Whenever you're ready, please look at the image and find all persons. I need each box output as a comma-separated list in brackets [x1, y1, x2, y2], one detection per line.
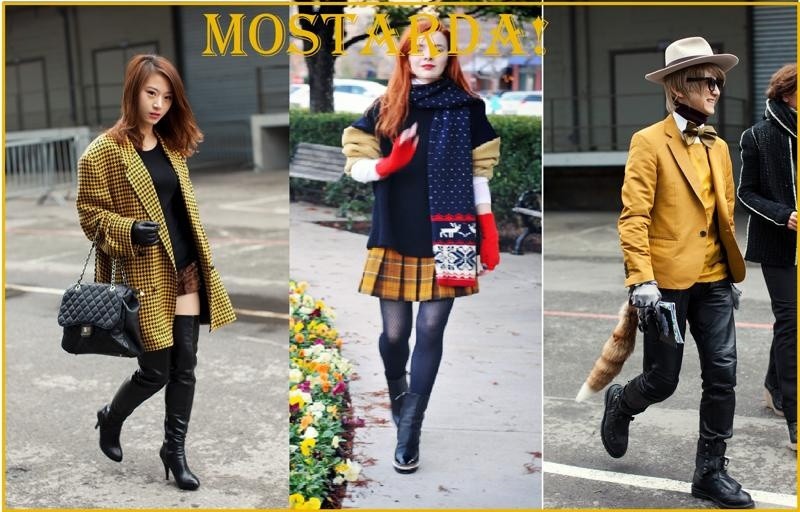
[466, 74, 481, 95]
[600, 35, 757, 508]
[339, 16, 504, 475]
[75, 51, 239, 492]
[736, 60, 797, 453]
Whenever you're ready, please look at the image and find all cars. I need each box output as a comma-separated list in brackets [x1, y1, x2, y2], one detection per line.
[290, 77, 542, 120]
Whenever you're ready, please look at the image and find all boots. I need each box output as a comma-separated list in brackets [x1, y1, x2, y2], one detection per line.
[692, 438, 754, 507]
[601, 376, 647, 460]
[381, 369, 429, 475]
[95, 313, 202, 492]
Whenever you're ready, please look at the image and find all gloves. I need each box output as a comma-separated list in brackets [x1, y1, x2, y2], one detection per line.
[132, 219, 166, 248]
[632, 282, 661, 333]
[474, 212, 501, 272]
[377, 130, 418, 179]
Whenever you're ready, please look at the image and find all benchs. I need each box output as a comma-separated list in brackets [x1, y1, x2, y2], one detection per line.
[289, 139, 351, 209]
[509, 189, 542, 251]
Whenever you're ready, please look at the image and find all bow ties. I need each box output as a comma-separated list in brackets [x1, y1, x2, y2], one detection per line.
[682, 120, 718, 149]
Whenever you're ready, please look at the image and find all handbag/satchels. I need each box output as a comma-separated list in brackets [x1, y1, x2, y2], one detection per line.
[57, 282, 145, 358]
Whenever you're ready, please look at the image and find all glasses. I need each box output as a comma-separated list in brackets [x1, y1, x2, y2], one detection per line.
[685, 74, 725, 91]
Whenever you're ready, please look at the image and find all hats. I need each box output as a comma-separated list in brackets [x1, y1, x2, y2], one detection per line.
[645, 35, 739, 84]
